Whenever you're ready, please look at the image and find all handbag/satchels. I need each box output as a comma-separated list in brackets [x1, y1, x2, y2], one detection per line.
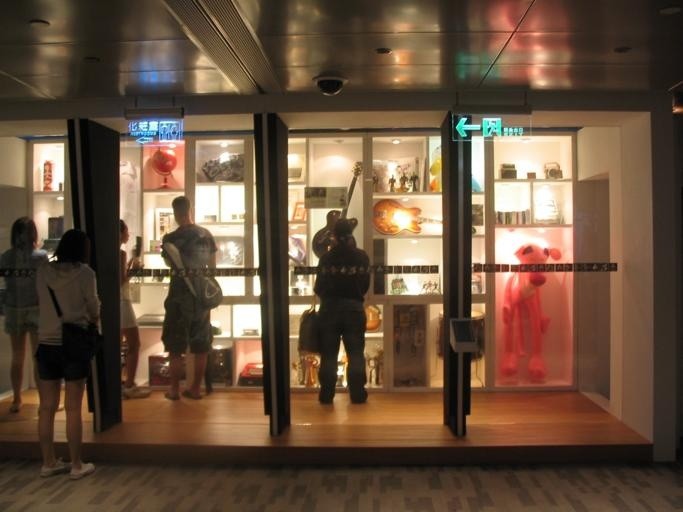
[297, 308, 321, 355]
[198, 273, 225, 309]
[59, 321, 101, 360]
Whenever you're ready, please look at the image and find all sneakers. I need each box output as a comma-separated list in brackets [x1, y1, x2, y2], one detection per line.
[121, 385, 152, 401]
[69, 463, 95, 480]
[39, 460, 70, 478]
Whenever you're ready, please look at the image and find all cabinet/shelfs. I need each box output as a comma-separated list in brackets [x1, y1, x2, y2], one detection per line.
[307, 136, 441, 301]
[246, 138, 313, 302]
[368, 131, 491, 396]
[116, 140, 143, 303]
[287, 299, 446, 394]
[488, 130, 577, 393]
[188, 138, 252, 300]
[141, 143, 196, 285]
[135, 285, 265, 395]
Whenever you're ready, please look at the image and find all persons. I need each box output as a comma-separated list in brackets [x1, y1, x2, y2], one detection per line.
[0, 216, 50, 415]
[311, 218, 370, 403]
[32, 227, 102, 480]
[118, 218, 153, 396]
[160, 196, 218, 402]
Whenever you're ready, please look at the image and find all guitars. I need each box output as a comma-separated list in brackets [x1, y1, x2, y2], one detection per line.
[365, 304, 380, 329]
[312, 162, 363, 258]
[371, 199, 476, 237]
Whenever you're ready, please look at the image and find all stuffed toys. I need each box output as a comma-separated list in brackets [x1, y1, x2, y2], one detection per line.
[500, 234, 561, 380]
